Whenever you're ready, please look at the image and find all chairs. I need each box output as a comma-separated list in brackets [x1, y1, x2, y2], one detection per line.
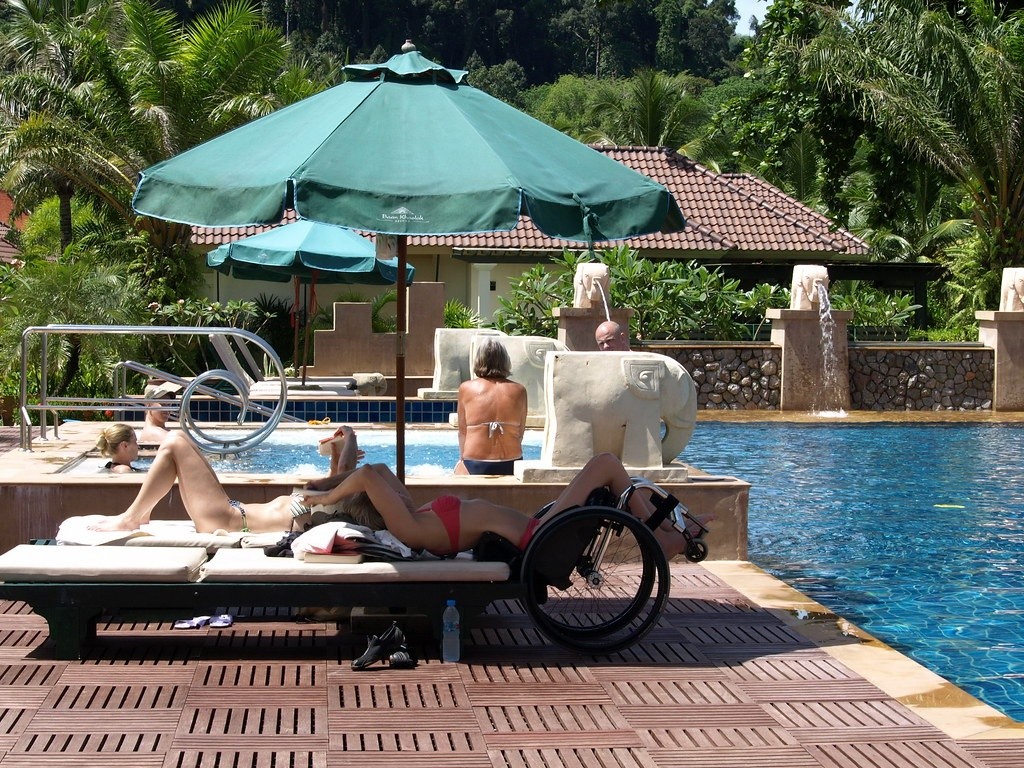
[231, 327, 357, 384]
[209, 332, 357, 396]
[216, 332, 348, 391]
[220, 333, 351, 388]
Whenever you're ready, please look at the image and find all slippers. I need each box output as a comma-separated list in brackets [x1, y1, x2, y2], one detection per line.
[209, 614, 232, 627]
[174, 616, 210, 628]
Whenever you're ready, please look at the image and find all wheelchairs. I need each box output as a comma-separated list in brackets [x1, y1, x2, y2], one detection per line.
[475, 478, 710, 652]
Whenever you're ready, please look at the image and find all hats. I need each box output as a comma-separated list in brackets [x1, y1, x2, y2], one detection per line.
[145, 388, 176, 399]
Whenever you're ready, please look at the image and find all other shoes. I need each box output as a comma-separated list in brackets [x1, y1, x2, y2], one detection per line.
[350, 621, 405, 670]
[390, 651, 413, 668]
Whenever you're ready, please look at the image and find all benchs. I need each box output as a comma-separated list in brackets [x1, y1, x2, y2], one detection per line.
[0, 513, 510, 660]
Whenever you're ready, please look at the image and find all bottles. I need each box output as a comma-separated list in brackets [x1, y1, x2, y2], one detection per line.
[442, 600, 460, 662]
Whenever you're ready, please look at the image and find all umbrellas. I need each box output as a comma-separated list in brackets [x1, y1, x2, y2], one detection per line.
[208, 216, 422, 388]
[132, 37, 688, 484]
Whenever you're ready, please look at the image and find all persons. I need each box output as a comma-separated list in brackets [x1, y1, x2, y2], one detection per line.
[298, 449, 720, 557]
[86, 424, 365, 534]
[96, 422, 140, 474]
[595, 320, 634, 351]
[452, 337, 528, 475]
[138, 384, 176, 442]
